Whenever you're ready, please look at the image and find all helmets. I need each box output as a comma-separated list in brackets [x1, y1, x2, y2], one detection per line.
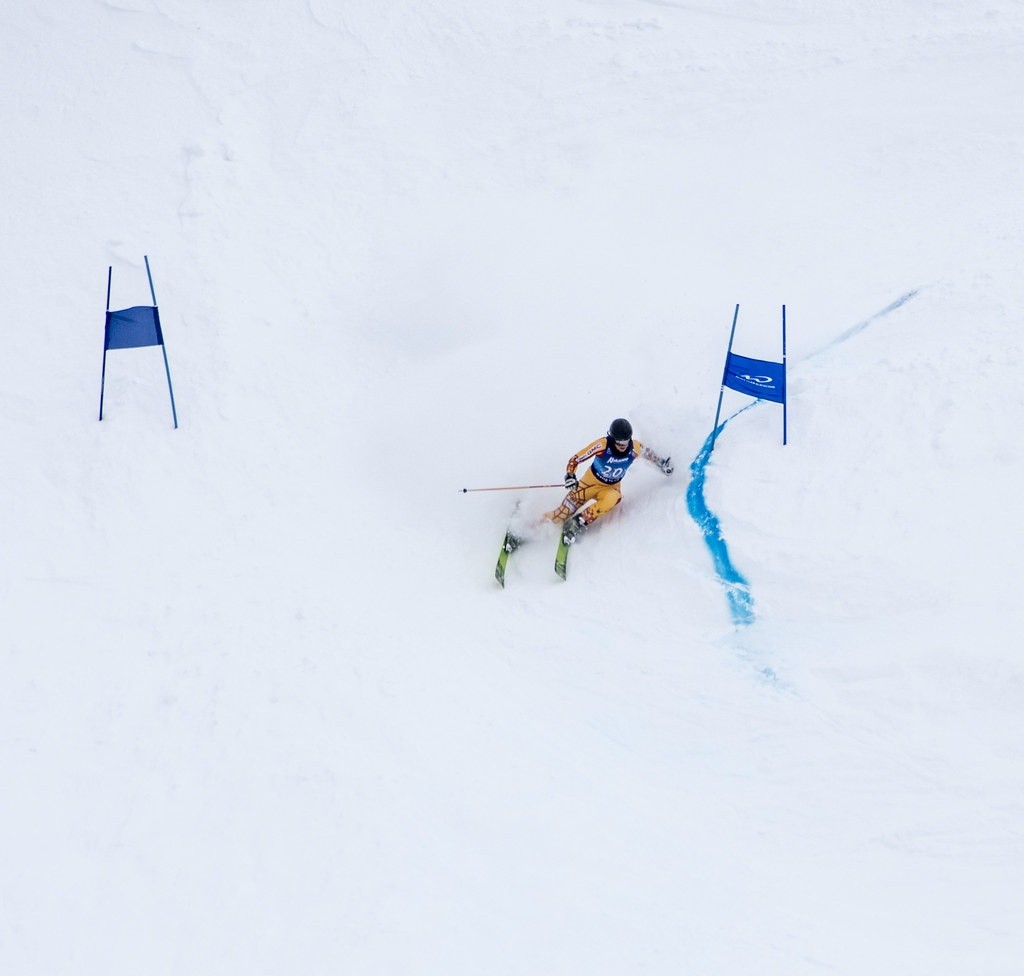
[607, 418, 633, 442]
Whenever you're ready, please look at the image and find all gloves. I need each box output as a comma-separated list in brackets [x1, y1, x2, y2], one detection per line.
[564, 474, 579, 493]
[661, 463, 674, 475]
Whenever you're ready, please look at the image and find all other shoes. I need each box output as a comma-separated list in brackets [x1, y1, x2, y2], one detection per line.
[509, 534, 518, 548]
[562, 521, 576, 546]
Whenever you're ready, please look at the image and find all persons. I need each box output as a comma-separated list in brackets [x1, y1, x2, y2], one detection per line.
[521, 418, 674, 556]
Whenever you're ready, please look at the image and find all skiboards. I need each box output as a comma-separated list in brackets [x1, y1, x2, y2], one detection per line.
[494, 499, 570, 590]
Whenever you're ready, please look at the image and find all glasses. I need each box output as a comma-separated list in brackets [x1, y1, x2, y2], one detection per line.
[615, 439, 630, 445]
